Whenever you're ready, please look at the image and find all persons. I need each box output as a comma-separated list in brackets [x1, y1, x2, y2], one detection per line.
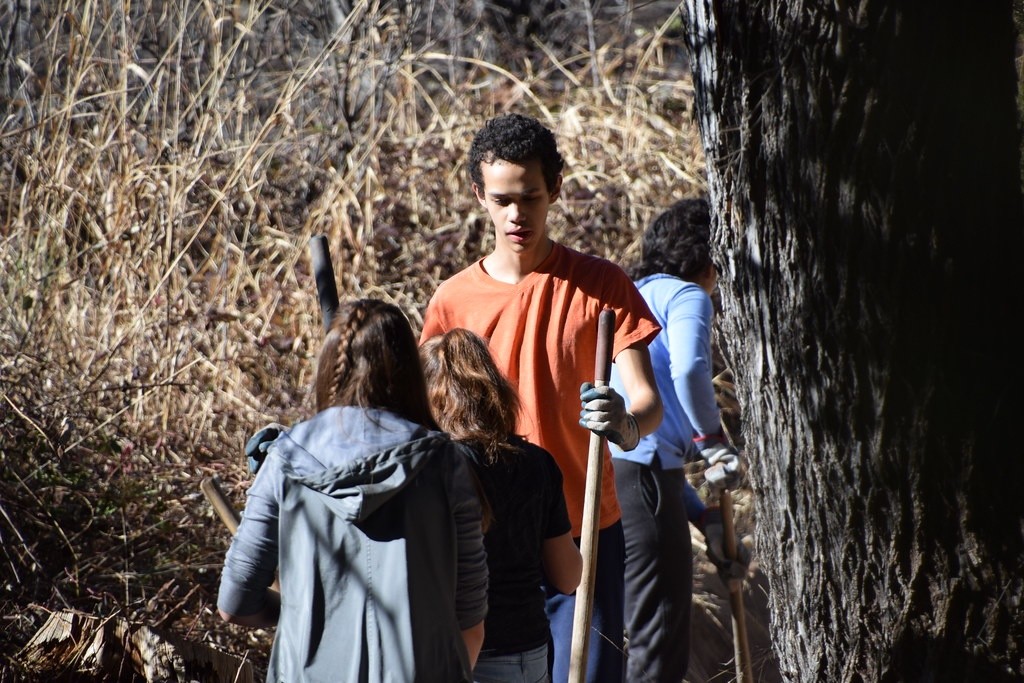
[215, 299, 488, 683]
[417, 114, 664, 683]
[418, 328, 584, 683]
[599, 198, 750, 683]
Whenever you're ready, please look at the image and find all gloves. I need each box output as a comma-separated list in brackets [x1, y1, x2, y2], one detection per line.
[245, 423, 292, 474]
[579, 382, 640, 451]
[692, 424, 744, 492]
